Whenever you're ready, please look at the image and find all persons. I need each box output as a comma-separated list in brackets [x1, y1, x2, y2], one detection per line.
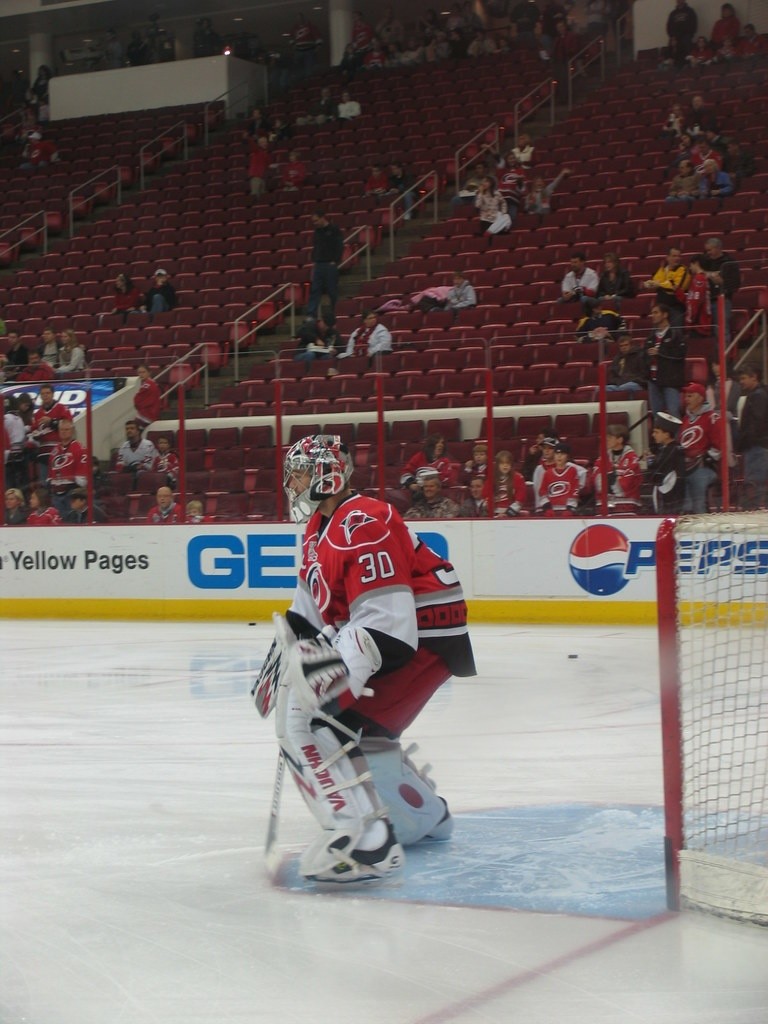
[0, 326, 214, 528]
[242, 87, 361, 198]
[295, 209, 477, 360]
[663, 96, 755, 202]
[252, 433, 477, 882]
[666, 0, 768, 63]
[95, 269, 177, 316]
[400, 357, 768, 520]
[361, 161, 418, 221]
[450, 132, 571, 238]
[558, 238, 742, 449]
[0, 12, 323, 169]
[337, 0, 632, 88]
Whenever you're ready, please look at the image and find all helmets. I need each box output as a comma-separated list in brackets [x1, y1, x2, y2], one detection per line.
[286, 434, 354, 524]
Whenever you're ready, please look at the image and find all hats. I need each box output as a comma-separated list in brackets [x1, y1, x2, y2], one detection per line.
[682, 381, 706, 399]
[654, 412, 682, 433]
[555, 443, 572, 454]
[544, 437, 560, 448]
[155, 269, 167, 276]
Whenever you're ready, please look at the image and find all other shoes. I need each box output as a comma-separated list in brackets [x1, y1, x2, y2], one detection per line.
[382, 796, 454, 842]
[304, 825, 404, 881]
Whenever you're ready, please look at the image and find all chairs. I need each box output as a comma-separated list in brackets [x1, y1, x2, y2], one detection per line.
[0, 32, 768, 526]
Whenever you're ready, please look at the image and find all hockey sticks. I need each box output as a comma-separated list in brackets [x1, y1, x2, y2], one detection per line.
[257, 744, 306, 889]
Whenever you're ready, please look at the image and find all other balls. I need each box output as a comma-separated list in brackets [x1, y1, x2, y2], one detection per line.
[568, 655, 578, 658]
[249, 623, 256, 625]
